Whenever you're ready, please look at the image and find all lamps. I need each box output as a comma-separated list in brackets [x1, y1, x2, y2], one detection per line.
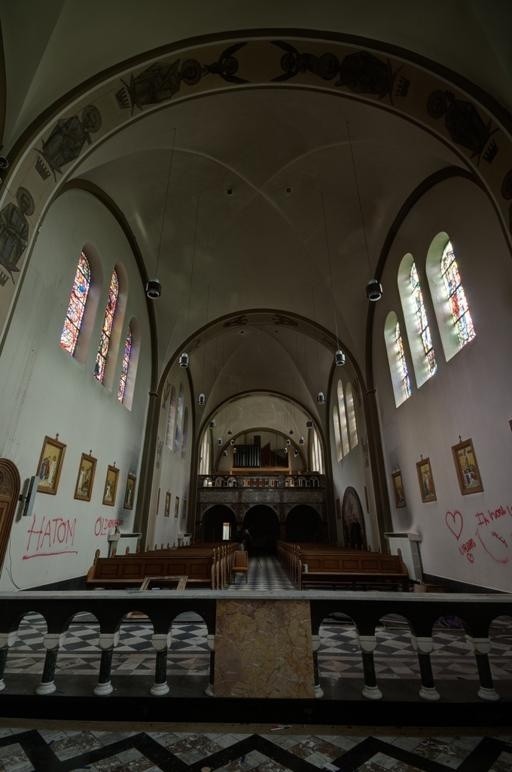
[344, 119, 384, 302]
[19, 476, 39, 517]
[179, 195, 200, 368]
[320, 188, 346, 368]
[146, 128, 176, 300]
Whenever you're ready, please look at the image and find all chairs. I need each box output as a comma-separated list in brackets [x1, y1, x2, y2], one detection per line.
[229, 550, 249, 585]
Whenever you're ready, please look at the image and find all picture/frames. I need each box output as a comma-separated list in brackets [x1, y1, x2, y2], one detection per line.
[392, 469, 406, 509]
[74, 452, 98, 501]
[415, 456, 437, 503]
[36, 435, 67, 496]
[124, 473, 137, 511]
[101, 464, 120, 507]
[451, 437, 484, 496]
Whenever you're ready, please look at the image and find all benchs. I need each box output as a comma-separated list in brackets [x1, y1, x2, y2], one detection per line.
[84, 539, 244, 591]
[278, 539, 413, 591]
